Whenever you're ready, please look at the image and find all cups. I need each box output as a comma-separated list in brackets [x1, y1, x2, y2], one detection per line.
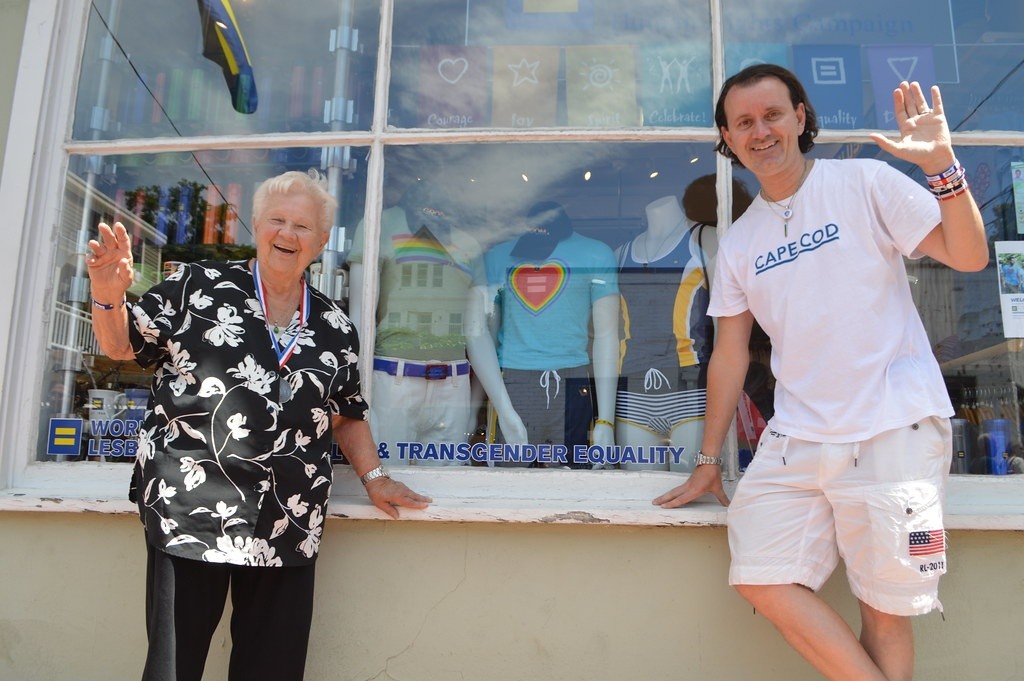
[87, 389, 127, 432]
[112, 183, 243, 262]
[950, 419, 971, 474]
[982, 419, 1011, 475]
[119, 65, 325, 123]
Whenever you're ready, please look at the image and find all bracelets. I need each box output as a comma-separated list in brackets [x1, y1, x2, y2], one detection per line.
[91, 296, 126, 311]
[925, 158, 969, 202]
[595, 420, 615, 432]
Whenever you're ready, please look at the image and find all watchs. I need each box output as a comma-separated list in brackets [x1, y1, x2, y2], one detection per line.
[359, 464, 389, 485]
[693, 449, 724, 467]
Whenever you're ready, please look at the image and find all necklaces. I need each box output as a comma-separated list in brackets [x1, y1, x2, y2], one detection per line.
[643, 218, 683, 268]
[761, 159, 809, 237]
[270, 318, 283, 334]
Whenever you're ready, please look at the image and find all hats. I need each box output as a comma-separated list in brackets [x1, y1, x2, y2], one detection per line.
[398, 177, 451, 247]
[510, 201, 570, 262]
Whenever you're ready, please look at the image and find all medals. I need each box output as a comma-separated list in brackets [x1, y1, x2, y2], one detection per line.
[279, 377, 292, 403]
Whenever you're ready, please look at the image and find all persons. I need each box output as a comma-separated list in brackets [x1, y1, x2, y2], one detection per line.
[613, 195, 718, 472]
[460, 201, 620, 470]
[83, 169, 433, 681]
[650, 63, 991, 681]
[346, 179, 529, 467]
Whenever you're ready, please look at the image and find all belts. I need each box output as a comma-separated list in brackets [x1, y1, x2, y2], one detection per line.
[373, 358, 470, 380]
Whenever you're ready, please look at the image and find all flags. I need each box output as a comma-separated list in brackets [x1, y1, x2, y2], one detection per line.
[197, 0, 258, 115]
